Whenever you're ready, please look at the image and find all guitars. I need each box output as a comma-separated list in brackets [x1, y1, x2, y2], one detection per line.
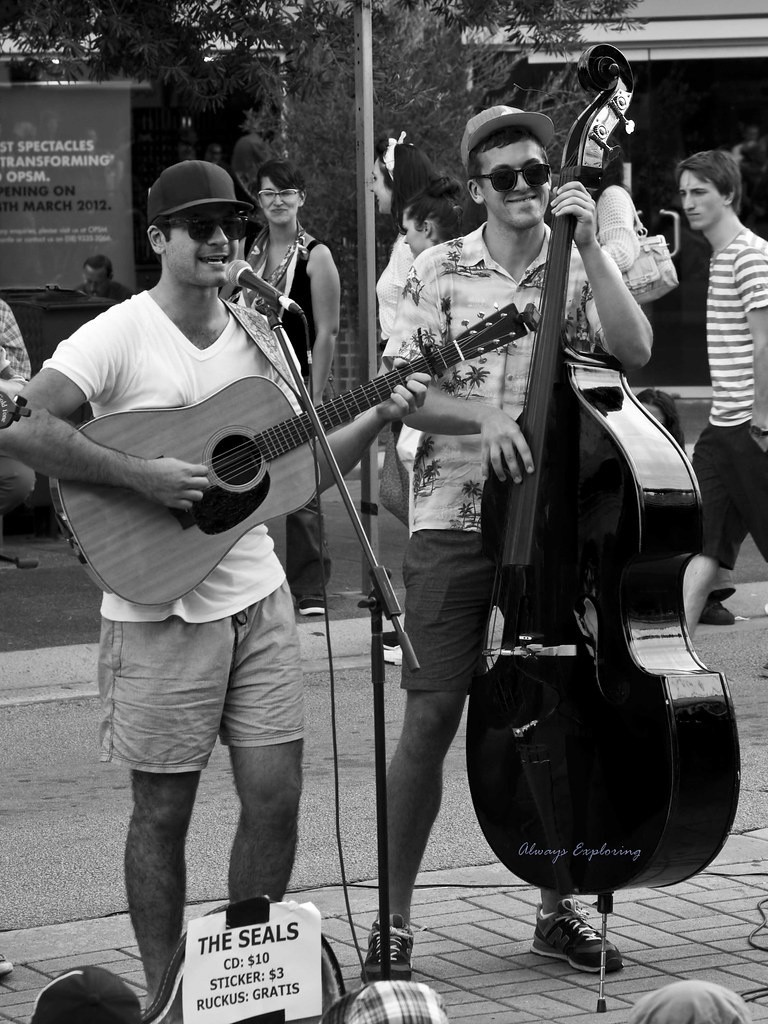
[50, 298, 529, 609]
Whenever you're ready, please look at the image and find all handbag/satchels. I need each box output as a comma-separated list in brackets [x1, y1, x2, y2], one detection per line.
[593, 185, 680, 305]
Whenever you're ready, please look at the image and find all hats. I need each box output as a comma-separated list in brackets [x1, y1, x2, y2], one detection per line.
[459, 105, 554, 168]
[145, 159, 254, 226]
[319, 980, 447, 1024]
[30, 965, 141, 1023]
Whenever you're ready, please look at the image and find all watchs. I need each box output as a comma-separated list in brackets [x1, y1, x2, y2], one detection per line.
[748, 423, 768, 438]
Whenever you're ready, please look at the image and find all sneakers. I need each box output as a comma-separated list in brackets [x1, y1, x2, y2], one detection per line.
[362, 914, 414, 985]
[530, 899, 624, 973]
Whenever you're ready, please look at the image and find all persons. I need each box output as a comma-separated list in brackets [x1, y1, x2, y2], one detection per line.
[319, 980, 449, 1024]
[636, 389, 736, 625]
[178, 130, 284, 188]
[627, 980, 752, 1024]
[669, 119, 768, 385]
[580, 136, 678, 305]
[220, 159, 341, 616]
[676, 150, 768, 642]
[0, 298, 36, 515]
[0, 954, 14, 975]
[368, 137, 466, 666]
[360, 105, 653, 981]
[0, 159, 431, 1024]
[77, 255, 132, 302]
[30, 965, 141, 1024]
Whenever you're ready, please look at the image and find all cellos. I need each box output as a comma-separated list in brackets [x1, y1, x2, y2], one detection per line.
[466, 43, 744, 1011]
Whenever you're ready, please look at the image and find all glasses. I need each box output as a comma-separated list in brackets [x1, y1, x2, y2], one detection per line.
[470, 162, 550, 192]
[258, 188, 300, 200]
[160, 213, 248, 241]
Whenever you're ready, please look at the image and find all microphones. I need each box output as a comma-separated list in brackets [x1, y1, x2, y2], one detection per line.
[226, 260, 305, 317]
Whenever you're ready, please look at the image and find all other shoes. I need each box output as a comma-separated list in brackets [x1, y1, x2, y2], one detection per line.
[299, 600, 326, 615]
[702, 599, 735, 626]
